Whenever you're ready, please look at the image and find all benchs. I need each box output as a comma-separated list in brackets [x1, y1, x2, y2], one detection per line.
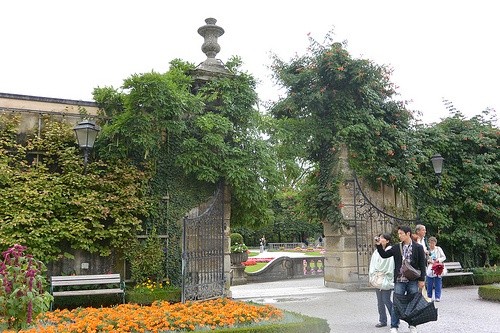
[442, 261, 475, 285]
[49, 273, 127, 313]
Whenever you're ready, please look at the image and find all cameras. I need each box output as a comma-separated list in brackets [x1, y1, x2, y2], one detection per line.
[374, 237, 380, 242]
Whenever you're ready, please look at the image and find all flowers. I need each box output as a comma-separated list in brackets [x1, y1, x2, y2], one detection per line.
[431, 261, 444, 277]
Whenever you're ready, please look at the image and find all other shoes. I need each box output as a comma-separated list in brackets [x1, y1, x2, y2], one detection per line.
[410, 326, 417, 333]
[435, 298, 440, 302]
[391, 328, 399, 333]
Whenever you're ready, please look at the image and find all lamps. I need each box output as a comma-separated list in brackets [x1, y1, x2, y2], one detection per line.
[430, 152, 445, 178]
[72, 119, 100, 175]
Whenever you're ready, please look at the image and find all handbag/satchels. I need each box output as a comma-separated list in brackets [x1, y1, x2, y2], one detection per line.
[370, 271, 390, 291]
[400, 242, 421, 281]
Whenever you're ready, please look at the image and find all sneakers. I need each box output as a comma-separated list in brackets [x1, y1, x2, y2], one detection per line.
[375, 322, 387, 327]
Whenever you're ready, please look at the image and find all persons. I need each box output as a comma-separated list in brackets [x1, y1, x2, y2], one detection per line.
[369, 233, 395, 327]
[411, 224, 429, 258]
[425, 237, 447, 302]
[374, 225, 426, 333]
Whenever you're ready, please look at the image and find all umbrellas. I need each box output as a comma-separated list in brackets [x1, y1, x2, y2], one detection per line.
[431, 262, 444, 275]
[393, 288, 438, 328]
